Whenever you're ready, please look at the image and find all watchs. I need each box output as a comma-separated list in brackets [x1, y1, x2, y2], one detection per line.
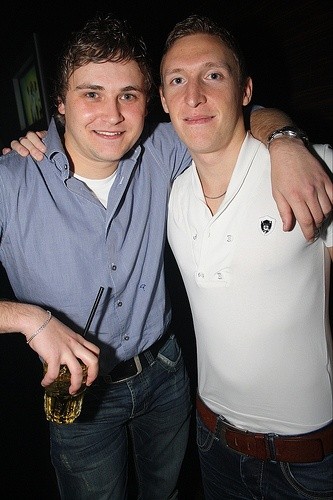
[266, 126, 310, 149]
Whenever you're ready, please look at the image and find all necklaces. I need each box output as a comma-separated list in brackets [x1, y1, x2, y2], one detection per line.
[204, 192, 226, 199]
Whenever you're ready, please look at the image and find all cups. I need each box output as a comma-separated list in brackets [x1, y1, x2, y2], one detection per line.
[43, 361, 89, 424]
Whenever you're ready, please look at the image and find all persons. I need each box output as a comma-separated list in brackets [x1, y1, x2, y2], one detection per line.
[0, 16, 333, 500]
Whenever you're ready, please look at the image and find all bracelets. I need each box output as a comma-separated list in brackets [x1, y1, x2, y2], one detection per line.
[27, 311, 51, 344]
